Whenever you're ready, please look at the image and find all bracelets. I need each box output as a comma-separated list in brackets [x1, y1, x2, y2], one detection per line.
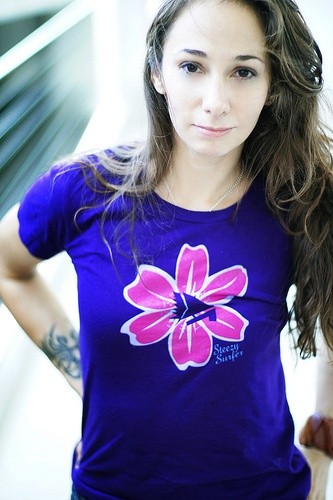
[297, 414, 333, 458]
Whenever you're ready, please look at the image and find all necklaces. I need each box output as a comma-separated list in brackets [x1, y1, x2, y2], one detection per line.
[152, 157, 247, 213]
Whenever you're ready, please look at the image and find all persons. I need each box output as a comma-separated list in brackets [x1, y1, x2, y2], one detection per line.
[0, 0, 333, 500]
